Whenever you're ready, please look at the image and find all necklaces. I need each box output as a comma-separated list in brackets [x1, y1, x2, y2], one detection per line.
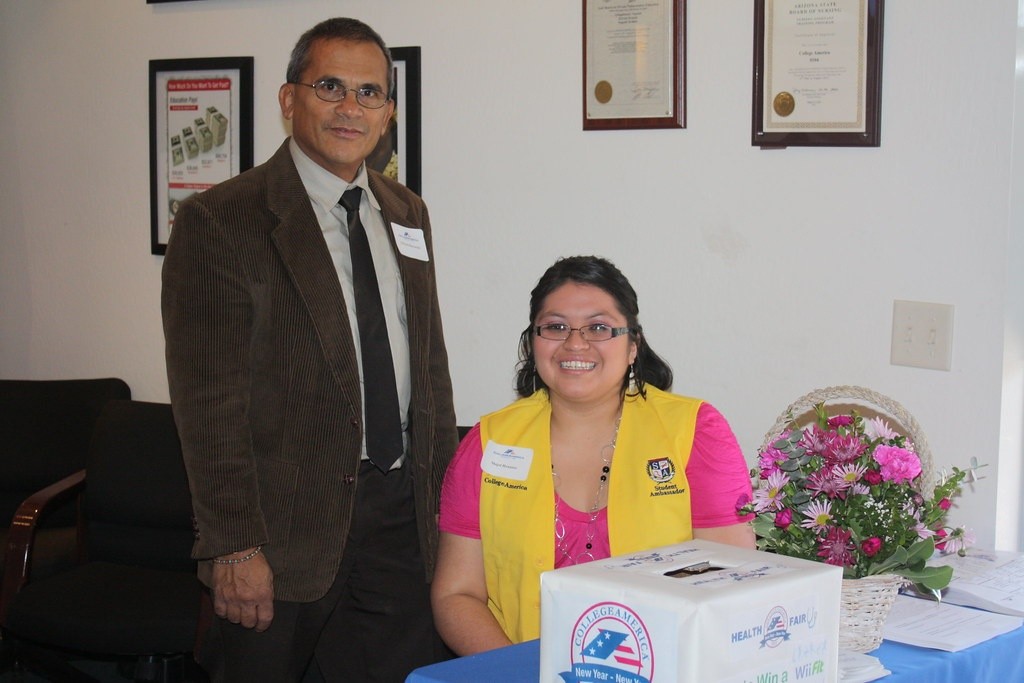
[551, 415, 621, 565]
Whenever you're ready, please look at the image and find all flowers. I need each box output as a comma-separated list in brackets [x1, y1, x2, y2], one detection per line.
[732, 397, 993, 604]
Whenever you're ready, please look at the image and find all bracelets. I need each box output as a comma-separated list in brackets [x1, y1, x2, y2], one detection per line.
[213, 546, 262, 564]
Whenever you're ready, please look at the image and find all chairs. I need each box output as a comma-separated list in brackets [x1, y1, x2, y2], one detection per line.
[0, 375, 132, 582]
[0, 396, 241, 683]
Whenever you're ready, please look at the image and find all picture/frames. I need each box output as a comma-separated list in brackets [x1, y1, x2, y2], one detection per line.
[365, 45, 422, 201]
[750, 0, 884, 150]
[147, 55, 256, 257]
[579, 0, 687, 130]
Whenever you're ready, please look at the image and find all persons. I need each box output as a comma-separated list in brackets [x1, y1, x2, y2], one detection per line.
[430, 255, 755, 657]
[157, 18, 461, 682]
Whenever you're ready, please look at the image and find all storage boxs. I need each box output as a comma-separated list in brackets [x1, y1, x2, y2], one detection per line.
[536, 539, 847, 682]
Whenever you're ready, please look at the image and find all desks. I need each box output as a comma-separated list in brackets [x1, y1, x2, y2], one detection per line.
[405, 542, 1023, 682]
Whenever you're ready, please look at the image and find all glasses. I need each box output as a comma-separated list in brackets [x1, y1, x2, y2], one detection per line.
[287, 78, 392, 109]
[530, 322, 630, 342]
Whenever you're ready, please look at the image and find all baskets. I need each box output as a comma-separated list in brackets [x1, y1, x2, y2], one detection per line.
[759, 384, 931, 653]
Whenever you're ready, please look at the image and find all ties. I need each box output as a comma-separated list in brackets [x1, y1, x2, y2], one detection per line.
[338, 186, 404, 475]
